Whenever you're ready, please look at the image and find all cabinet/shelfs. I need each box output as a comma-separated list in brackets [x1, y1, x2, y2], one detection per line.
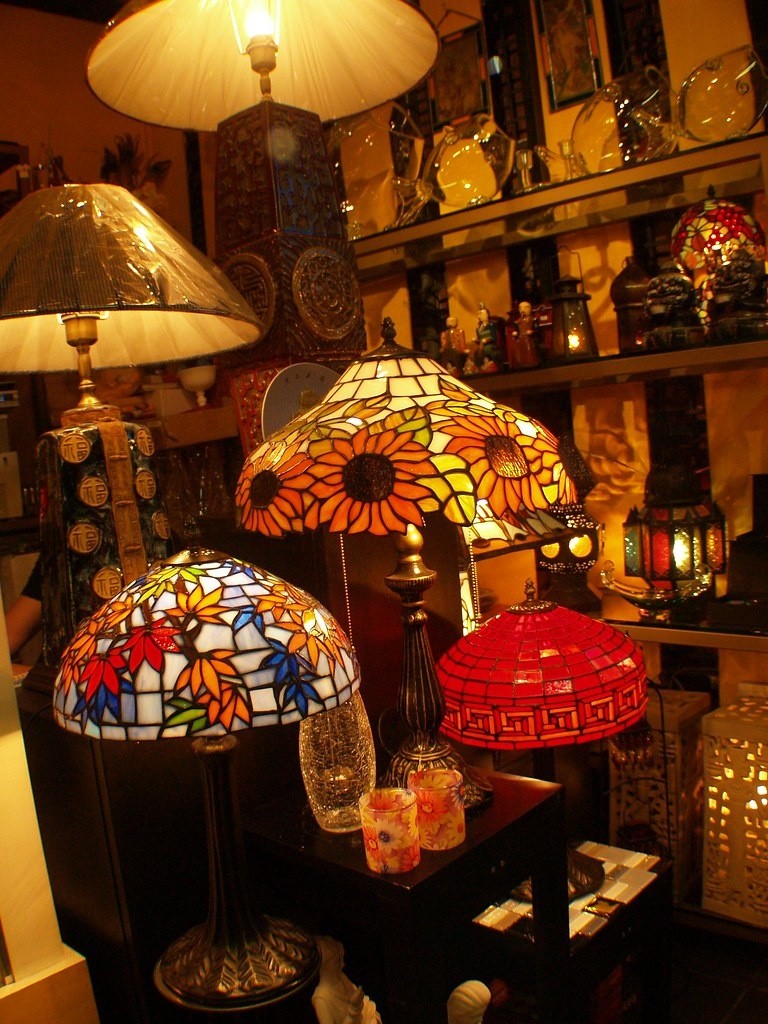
[19, 132, 768, 1024]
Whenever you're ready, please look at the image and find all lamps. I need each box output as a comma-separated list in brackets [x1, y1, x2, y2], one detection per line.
[599, 689, 712, 900]
[701, 680, 768, 930]
[537, 430, 605, 612]
[233, 318, 581, 804]
[436, 580, 651, 904]
[86, 0, 444, 461]
[623, 434, 727, 624]
[0, 184, 265, 673]
[48, 538, 363, 1024]
[670, 183, 768, 341]
[551, 274, 601, 362]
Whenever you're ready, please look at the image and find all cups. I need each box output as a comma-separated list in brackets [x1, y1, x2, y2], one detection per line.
[411, 769, 466, 851]
[358, 788, 420, 874]
